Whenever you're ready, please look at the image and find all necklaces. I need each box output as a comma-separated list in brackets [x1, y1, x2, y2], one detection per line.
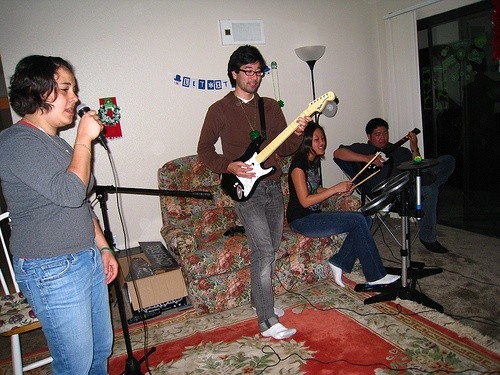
[236, 89, 260, 140]
[22, 118, 70, 153]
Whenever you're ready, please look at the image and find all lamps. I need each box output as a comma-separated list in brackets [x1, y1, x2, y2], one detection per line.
[295, 45, 339, 187]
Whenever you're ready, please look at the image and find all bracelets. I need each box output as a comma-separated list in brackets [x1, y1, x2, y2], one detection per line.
[74, 143, 92, 155]
[100, 248, 115, 255]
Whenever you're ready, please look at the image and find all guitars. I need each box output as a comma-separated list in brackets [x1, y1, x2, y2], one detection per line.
[220, 90, 337, 203]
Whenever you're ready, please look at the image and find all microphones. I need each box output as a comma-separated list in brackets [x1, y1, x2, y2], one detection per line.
[75, 104, 109, 150]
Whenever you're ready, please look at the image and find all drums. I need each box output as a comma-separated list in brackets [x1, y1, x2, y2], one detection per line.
[360, 193, 391, 217]
[371, 171, 416, 197]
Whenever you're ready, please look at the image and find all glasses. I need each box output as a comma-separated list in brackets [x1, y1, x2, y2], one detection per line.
[238, 68, 264, 77]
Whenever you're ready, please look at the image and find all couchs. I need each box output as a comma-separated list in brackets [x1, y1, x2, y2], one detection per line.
[155, 147, 360, 313]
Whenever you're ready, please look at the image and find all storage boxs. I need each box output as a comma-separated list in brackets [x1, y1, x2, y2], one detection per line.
[115, 242, 189, 324]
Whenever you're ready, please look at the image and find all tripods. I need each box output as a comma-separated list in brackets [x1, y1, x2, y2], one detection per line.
[91, 186, 212, 374]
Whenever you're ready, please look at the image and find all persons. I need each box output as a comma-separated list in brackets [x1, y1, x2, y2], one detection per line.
[333, 118, 456, 253]
[0, 55, 120, 375]
[198, 45, 312, 340]
[286, 121, 401, 288]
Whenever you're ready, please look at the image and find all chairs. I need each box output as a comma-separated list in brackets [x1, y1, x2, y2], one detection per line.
[0, 212, 53, 375]
[333, 151, 424, 249]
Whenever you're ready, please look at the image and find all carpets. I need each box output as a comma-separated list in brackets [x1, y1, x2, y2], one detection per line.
[0, 268, 500, 375]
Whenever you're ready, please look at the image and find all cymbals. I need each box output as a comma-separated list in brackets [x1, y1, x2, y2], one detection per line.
[396, 159, 440, 170]
